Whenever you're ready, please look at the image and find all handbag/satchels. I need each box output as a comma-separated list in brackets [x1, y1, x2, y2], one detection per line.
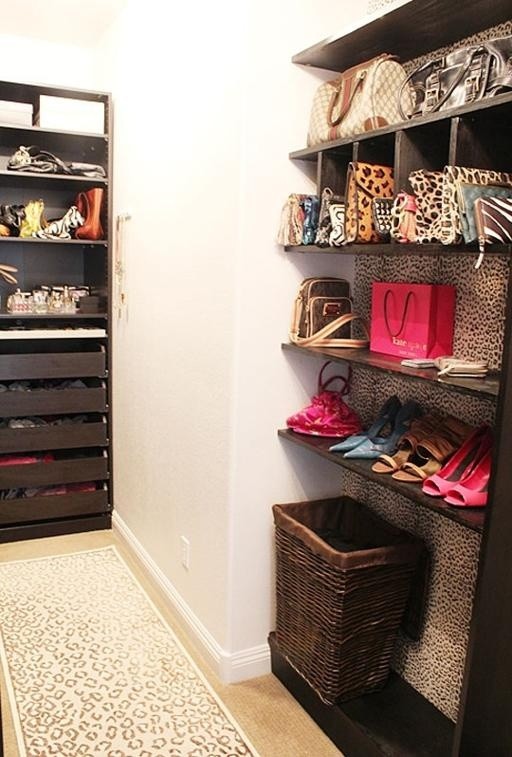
[288, 277, 369, 348]
[0, 188, 104, 241]
[286, 361, 361, 438]
[369, 282, 456, 360]
[396, 35, 512, 122]
[306, 53, 416, 147]
[7, 145, 106, 179]
[276, 162, 512, 271]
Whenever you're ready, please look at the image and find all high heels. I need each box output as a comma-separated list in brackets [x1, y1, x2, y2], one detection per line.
[328, 395, 402, 452]
[443, 448, 492, 507]
[343, 400, 419, 459]
[392, 416, 472, 482]
[372, 414, 437, 473]
[422, 424, 493, 497]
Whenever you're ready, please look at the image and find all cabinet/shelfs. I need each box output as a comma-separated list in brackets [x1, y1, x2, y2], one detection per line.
[268, 0, 512, 757]
[1, 78, 116, 547]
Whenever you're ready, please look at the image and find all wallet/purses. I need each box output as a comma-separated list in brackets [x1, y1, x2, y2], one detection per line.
[434, 356, 488, 379]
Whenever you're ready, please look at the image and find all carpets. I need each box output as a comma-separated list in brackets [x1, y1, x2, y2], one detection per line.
[1, 547, 258, 757]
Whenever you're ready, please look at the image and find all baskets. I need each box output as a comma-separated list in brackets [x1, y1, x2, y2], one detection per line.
[271, 496, 427, 700]
